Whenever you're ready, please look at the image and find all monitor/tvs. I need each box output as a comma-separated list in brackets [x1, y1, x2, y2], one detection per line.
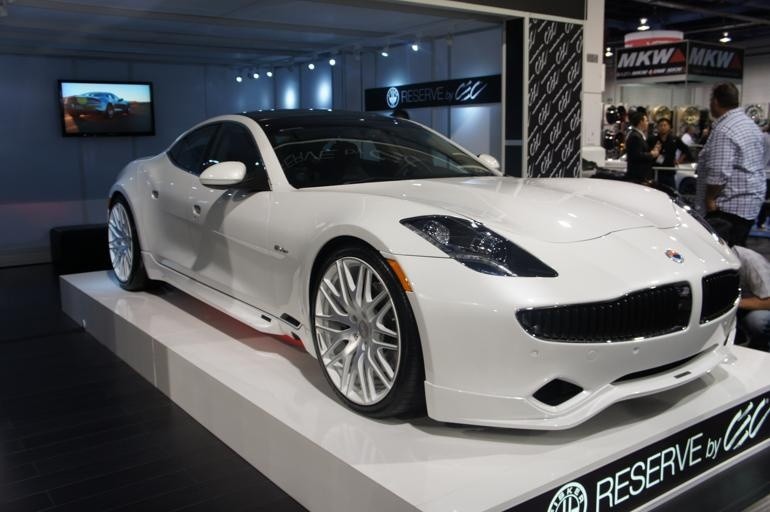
[57, 78, 156, 137]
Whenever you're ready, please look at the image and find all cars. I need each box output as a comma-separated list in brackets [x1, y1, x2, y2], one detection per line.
[581, 139, 770, 230]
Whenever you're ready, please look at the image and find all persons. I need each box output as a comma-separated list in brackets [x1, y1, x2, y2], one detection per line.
[758, 121, 770, 231]
[603, 105, 712, 188]
[695, 81, 767, 247]
[710, 217, 769, 350]
[377, 111, 439, 167]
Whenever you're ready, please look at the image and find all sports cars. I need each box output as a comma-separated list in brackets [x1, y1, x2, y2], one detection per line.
[66, 90, 152, 135]
[104, 105, 748, 438]
